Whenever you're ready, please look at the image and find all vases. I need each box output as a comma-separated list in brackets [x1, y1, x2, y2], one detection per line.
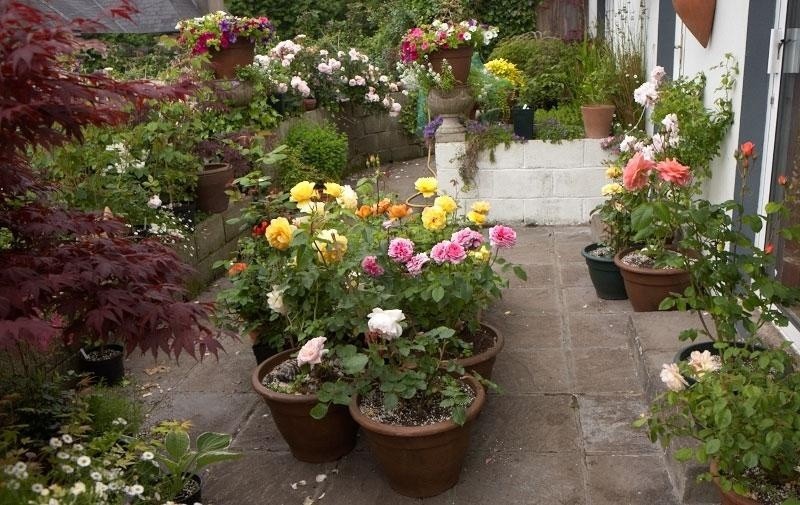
[207, 41, 256, 79]
[672, 340, 790, 435]
[248, 318, 506, 500]
[513, 104, 536, 137]
[80, 344, 125, 380]
[196, 162, 232, 213]
[704, 451, 799, 503]
[579, 237, 691, 314]
[427, 44, 474, 84]
[579, 103, 615, 137]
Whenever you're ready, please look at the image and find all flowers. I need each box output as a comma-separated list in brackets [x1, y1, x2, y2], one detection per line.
[296, 336, 330, 368]
[399, 20, 500, 58]
[778, 175, 787, 186]
[593, 57, 740, 252]
[660, 350, 720, 392]
[763, 244, 776, 255]
[740, 142, 756, 159]
[173, 10, 274, 56]
[256, 41, 405, 118]
[227, 178, 517, 280]
[623, 152, 691, 191]
[367, 307, 405, 341]
[267, 284, 292, 315]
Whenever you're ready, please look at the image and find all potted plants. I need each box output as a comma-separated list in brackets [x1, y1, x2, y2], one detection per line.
[146, 427, 242, 505]
